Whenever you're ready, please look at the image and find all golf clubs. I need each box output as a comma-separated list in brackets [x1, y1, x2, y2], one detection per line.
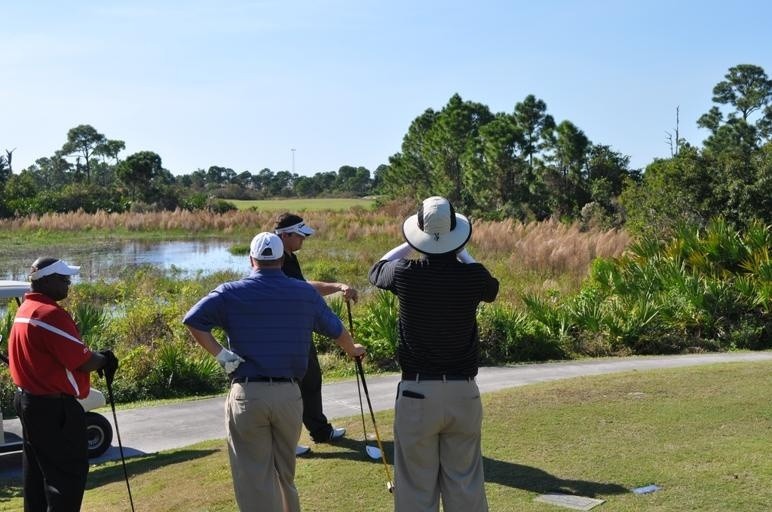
[346, 301, 382, 459]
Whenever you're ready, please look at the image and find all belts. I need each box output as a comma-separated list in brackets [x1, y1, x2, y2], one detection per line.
[234, 377, 298, 383]
[17, 386, 71, 399]
[402, 371, 474, 381]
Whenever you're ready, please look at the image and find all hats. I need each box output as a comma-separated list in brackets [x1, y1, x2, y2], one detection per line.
[28, 259, 80, 280]
[403, 195, 472, 255]
[250, 232, 284, 260]
[275, 220, 313, 236]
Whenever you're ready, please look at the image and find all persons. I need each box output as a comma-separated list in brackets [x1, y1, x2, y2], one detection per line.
[274, 211, 359, 457]
[9, 256, 119, 512]
[368, 196, 498, 512]
[181, 232, 368, 512]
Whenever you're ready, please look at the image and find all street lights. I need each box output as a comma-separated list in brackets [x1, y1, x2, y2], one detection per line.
[289, 147, 297, 171]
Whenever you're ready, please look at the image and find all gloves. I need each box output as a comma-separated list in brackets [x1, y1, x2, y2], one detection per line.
[215, 348, 245, 374]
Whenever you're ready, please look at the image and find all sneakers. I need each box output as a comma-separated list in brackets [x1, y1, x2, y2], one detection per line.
[296, 446, 310, 455]
[331, 428, 346, 440]
